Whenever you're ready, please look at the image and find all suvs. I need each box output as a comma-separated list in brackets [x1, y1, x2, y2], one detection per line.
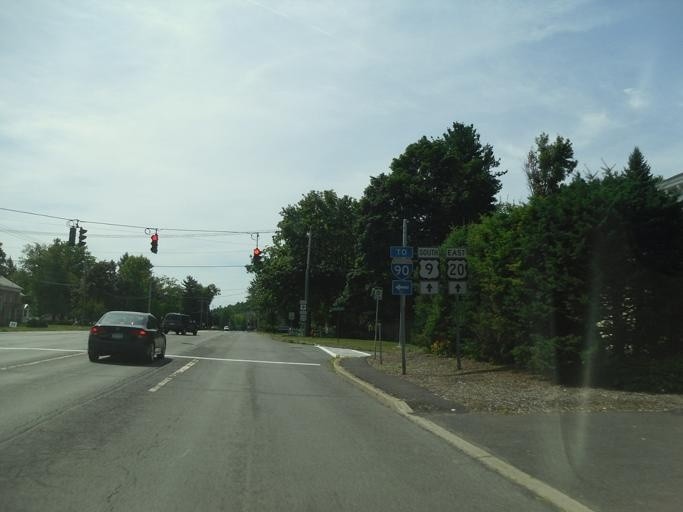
[160, 313, 198, 336]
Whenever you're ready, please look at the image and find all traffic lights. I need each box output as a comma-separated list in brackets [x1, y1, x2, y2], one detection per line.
[253, 248, 261, 265]
[78, 227, 87, 246]
[151, 233, 157, 254]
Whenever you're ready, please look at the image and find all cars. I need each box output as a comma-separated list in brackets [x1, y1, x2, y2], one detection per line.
[87, 310, 167, 363]
[223, 326, 229, 330]
